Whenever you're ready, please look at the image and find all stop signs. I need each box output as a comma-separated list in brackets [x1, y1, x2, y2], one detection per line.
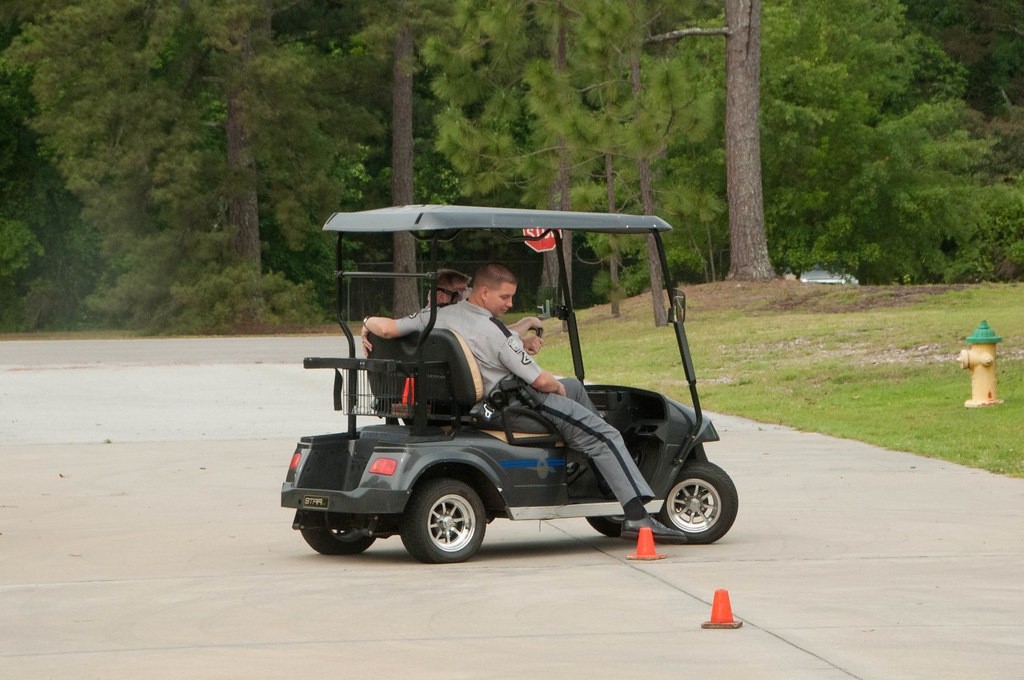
[522, 226, 562, 253]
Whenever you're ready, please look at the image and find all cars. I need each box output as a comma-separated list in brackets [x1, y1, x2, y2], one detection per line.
[798, 269, 847, 285]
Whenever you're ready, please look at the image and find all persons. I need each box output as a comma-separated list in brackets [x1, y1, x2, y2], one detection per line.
[398, 268, 544, 399]
[359, 263, 688, 544]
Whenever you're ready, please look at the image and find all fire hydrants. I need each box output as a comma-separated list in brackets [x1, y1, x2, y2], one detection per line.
[957, 319, 1005, 407]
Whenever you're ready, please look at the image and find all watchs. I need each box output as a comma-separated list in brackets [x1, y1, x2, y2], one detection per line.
[363, 315, 370, 333]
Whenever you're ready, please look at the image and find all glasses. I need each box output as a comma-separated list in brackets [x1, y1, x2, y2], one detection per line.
[437, 287, 472, 301]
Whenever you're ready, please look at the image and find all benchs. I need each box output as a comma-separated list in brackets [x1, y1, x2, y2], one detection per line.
[799, 265, 859, 285]
[365, 325, 563, 446]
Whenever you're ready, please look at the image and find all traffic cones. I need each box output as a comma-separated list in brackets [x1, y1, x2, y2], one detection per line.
[699, 588, 744, 628]
[625, 528, 666, 561]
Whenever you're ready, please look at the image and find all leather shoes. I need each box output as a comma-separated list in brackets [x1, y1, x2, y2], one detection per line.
[621, 514, 687, 544]
